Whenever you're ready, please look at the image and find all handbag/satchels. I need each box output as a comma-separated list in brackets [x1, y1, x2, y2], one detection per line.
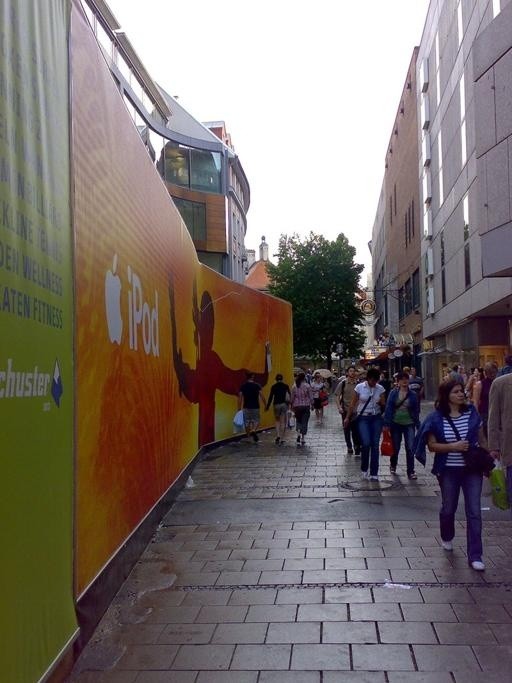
[489, 458, 510, 510]
[348, 395, 372, 427]
[380, 425, 395, 456]
[462, 446, 496, 474]
[284, 390, 292, 403]
[319, 390, 329, 407]
[232, 409, 244, 427]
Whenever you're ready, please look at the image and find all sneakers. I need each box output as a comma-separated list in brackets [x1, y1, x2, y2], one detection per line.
[348, 447, 361, 456]
[275, 434, 288, 444]
[362, 471, 379, 482]
[441, 538, 453, 551]
[297, 434, 307, 444]
[251, 430, 259, 442]
[470, 560, 486, 572]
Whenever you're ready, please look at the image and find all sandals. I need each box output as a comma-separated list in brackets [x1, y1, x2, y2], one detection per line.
[389, 464, 396, 475]
[407, 471, 418, 480]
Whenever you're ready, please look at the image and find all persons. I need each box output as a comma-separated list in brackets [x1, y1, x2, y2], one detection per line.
[289, 353, 512, 508]
[238, 370, 266, 442]
[265, 374, 291, 444]
[410, 374, 490, 572]
